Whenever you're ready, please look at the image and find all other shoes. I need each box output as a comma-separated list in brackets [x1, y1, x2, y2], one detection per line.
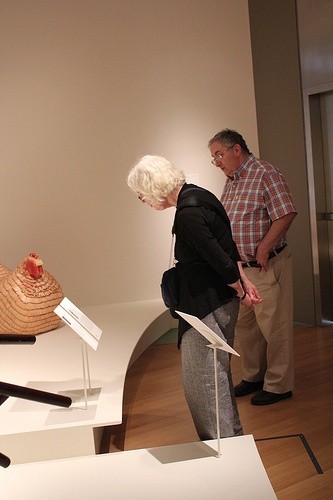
[251, 388, 293, 406]
[232, 379, 263, 397]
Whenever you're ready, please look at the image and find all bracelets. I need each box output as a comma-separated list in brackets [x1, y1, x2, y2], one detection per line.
[236, 292, 246, 301]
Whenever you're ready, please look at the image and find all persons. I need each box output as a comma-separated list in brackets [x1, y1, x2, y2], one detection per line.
[207, 129, 296, 405]
[125, 155, 264, 442]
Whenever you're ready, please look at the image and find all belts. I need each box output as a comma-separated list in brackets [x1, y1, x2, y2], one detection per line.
[241, 242, 287, 267]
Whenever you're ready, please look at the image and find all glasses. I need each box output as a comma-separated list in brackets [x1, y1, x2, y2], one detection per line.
[210, 144, 238, 165]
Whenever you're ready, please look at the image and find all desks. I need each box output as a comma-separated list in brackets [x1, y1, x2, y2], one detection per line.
[0, 299, 278, 500]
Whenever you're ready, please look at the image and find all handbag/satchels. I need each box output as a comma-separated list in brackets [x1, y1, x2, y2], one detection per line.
[161, 266, 178, 308]
